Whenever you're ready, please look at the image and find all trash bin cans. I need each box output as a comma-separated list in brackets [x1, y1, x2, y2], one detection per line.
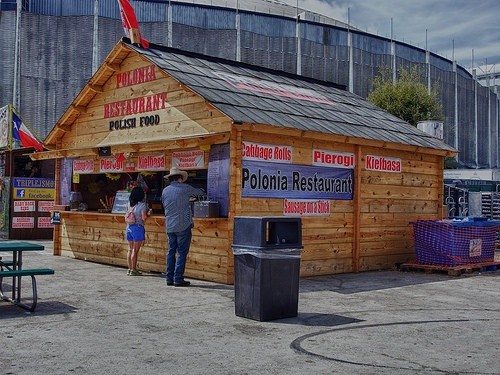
[231, 217, 304, 322]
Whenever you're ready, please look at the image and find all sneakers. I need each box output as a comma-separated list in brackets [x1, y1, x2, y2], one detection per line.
[126, 269, 142, 276]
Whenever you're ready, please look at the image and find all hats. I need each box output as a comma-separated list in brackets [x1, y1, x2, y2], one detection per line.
[164, 167, 188, 182]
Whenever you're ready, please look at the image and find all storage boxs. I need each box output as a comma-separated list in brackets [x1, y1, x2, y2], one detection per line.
[193, 200, 220, 218]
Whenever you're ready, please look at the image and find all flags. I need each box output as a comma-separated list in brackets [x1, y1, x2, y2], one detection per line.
[118, 0, 150, 49]
[11, 111, 43, 152]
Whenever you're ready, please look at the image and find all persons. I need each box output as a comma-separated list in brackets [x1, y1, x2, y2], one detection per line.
[126, 186, 151, 276]
[162, 167, 205, 287]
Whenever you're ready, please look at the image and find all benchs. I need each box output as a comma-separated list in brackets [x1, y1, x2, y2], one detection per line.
[0, 269, 54, 312]
[0, 261, 22, 291]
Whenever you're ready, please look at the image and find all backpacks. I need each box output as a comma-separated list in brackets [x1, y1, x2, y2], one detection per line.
[125, 203, 140, 224]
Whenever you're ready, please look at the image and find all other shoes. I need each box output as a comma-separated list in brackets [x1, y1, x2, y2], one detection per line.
[175, 281, 190, 287]
[167, 282, 174, 286]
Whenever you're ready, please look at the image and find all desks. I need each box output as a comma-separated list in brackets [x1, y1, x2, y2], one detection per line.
[0, 241, 44, 303]
